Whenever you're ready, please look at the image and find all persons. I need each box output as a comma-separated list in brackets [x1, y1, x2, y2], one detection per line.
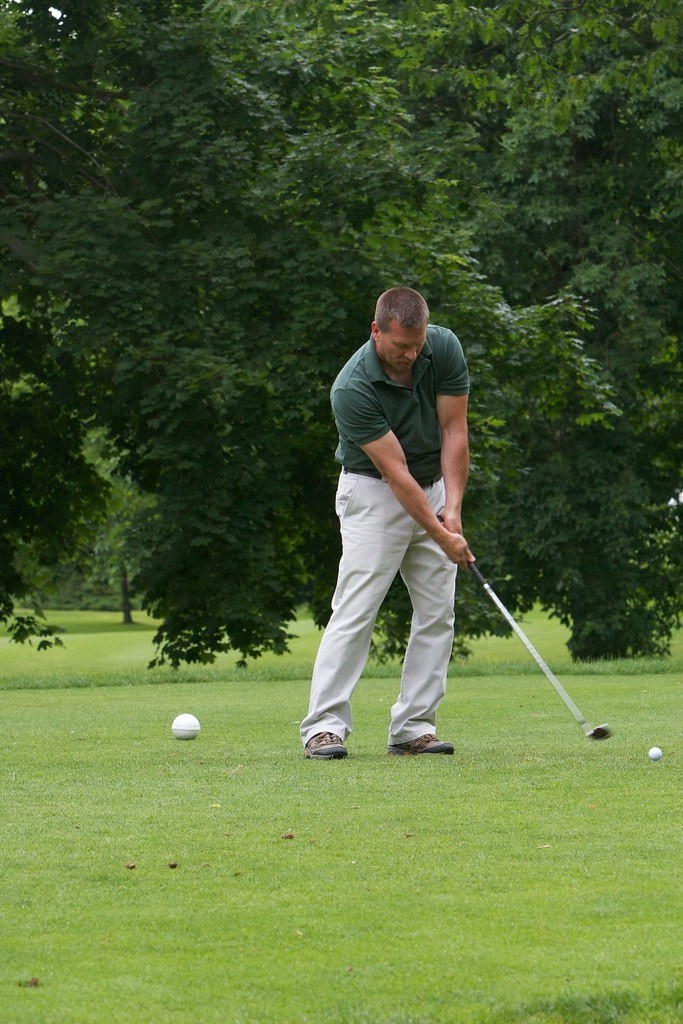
[301, 286, 470, 760]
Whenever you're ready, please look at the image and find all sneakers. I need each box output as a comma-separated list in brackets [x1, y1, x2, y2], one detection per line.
[388, 734, 454, 755]
[304, 731, 348, 760]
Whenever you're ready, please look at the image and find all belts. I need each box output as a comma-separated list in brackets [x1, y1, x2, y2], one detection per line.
[344, 467, 443, 489]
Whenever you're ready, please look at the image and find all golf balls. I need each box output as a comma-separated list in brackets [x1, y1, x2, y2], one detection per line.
[649, 747, 662, 761]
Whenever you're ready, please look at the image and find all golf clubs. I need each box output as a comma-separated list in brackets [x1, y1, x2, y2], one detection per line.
[437, 514, 611, 740]
